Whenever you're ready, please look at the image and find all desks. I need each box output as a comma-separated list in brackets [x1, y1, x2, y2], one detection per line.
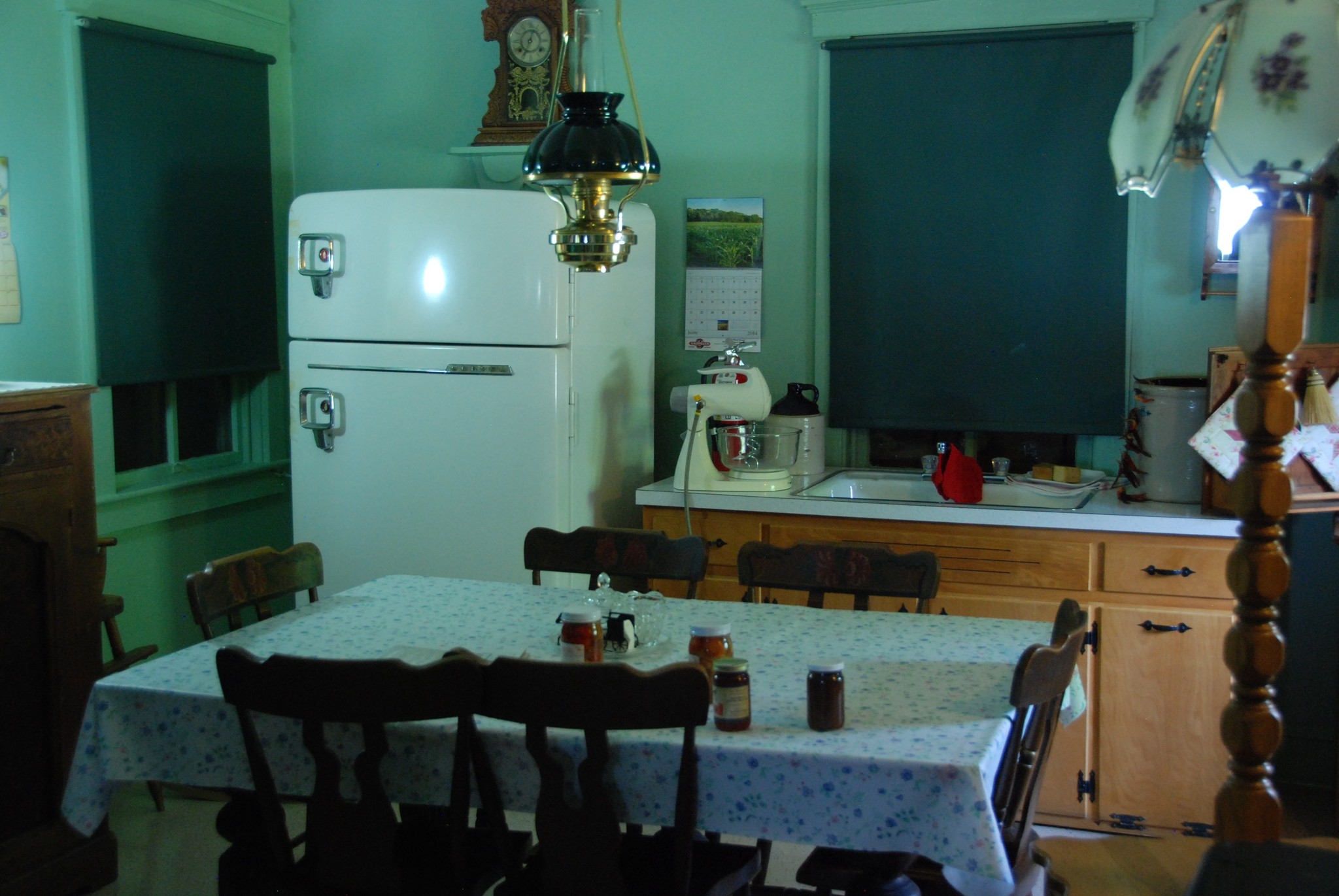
[59, 569, 1087, 896]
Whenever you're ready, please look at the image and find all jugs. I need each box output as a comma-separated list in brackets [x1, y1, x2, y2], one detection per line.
[579, 572, 640, 619]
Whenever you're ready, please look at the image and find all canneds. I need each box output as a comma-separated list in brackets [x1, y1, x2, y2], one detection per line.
[560, 604, 604, 662]
[807, 661, 845, 732]
[689, 620, 733, 701]
[712, 658, 751, 732]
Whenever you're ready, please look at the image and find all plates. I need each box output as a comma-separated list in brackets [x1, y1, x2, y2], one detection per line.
[1026, 465, 1106, 490]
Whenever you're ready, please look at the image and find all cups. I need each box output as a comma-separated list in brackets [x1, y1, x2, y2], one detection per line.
[627, 590, 666, 646]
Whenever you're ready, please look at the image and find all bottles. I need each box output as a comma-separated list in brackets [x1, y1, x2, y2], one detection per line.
[763, 382, 825, 477]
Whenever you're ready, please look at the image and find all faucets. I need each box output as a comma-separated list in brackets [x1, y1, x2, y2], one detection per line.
[936, 437, 978, 460]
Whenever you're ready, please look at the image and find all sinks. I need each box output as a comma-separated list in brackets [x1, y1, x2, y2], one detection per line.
[790, 467, 1104, 511]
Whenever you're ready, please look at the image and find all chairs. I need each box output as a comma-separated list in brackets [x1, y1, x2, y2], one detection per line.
[522, 524, 717, 599]
[213, 644, 536, 896]
[186, 540, 326, 640]
[439, 644, 774, 896]
[793, 595, 1091, 896]
[734, 537, 941, 616]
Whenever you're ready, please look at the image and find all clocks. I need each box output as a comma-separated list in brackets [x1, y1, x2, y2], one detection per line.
[505, 16, 552, 70]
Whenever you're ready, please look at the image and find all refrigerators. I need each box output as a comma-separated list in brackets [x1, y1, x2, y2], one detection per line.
[283, 186, 657, 609]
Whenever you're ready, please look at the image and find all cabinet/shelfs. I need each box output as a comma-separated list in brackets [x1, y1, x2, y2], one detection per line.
[0, 381, 95, 896]
[927, 592, 1238, 833]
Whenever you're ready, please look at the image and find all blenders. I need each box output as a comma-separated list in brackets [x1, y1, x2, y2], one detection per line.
[670, 366, 801, 493]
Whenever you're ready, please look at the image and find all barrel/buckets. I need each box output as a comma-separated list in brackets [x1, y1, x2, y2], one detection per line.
[1131, 376, 1207, 505]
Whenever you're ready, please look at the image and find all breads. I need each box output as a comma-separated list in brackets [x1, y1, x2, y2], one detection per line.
[1033, 463, 1054, 481]
[1054, 466, 1081, 483]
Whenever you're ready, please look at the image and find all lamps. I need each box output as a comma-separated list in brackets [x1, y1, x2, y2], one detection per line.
[520, 0, 663, 273]
